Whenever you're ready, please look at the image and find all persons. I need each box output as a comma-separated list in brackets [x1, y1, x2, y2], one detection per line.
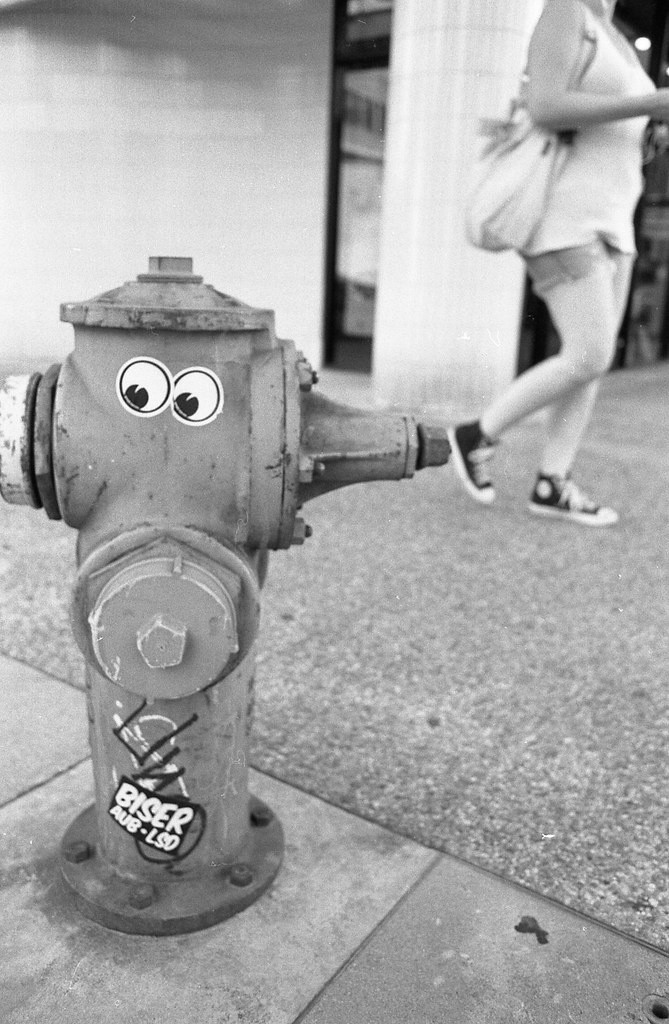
[446, 0, 668, 523]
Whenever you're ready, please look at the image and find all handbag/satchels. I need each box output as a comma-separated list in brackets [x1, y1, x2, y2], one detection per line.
[466, 5, 596, 251]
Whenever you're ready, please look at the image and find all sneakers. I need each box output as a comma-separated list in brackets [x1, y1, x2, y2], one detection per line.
[529, 475, 619, 528]
[449, 421, 497, 506]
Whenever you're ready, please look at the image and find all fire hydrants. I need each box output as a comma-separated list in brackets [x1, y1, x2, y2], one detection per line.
[1, 258, 451, 937]
